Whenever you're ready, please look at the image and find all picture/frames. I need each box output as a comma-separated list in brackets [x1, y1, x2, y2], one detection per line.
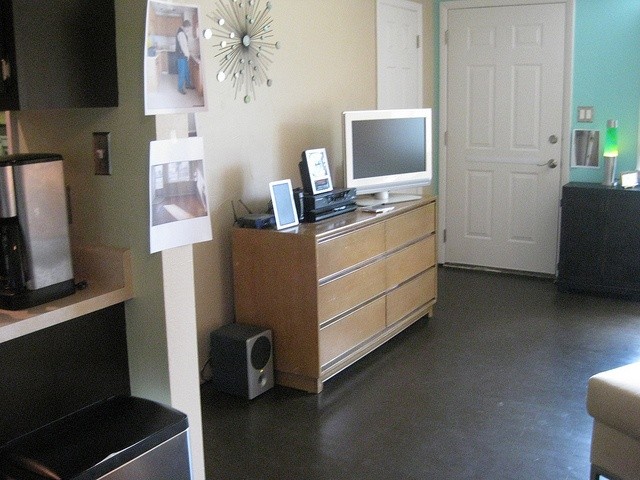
[268, 178, 299, 231]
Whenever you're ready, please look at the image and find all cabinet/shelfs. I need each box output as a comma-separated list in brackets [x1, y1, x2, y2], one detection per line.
[556, 181, 640, 296]
[231, 194, 440, 393]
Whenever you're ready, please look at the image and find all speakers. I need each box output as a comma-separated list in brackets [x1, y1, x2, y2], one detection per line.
[200, 322, 276, 401]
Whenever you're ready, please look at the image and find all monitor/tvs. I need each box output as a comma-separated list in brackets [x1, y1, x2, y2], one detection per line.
[341, 108, 433, 206]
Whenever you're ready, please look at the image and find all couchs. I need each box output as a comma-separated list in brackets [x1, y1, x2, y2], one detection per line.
[585, 359, 640, 480]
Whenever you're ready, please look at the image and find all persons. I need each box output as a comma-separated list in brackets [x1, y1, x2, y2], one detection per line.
[175, 20, 196, 94]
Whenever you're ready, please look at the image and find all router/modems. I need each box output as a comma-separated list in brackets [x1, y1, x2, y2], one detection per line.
[231, 199, 276, 229]
